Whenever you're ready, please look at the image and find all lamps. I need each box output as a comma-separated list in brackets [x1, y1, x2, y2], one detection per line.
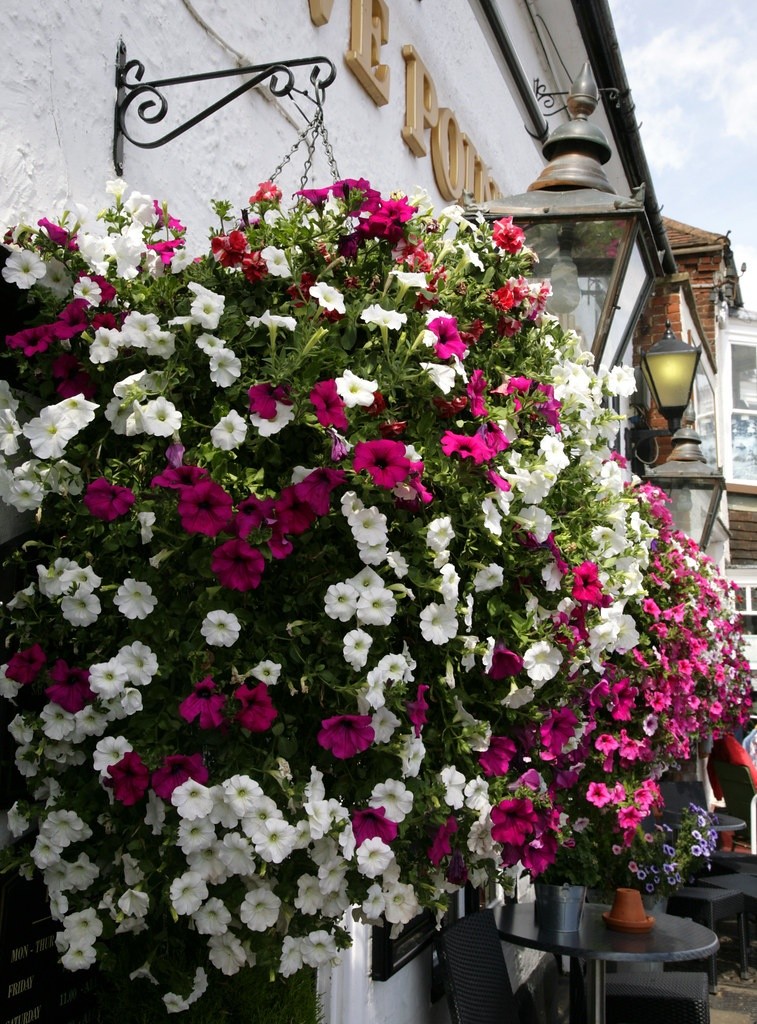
[461, 63, 679, 400]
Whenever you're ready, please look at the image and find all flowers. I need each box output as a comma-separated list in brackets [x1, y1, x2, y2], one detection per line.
[1, 177, 755, 1024]
